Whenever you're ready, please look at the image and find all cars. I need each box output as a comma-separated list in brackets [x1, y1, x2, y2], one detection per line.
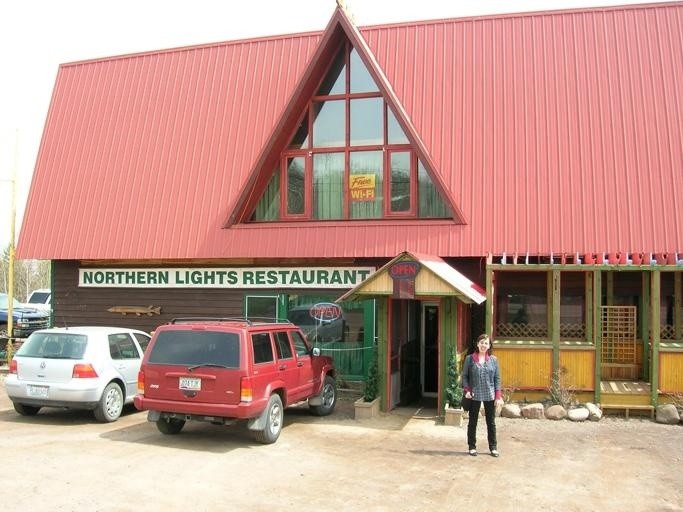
[2, 315, 160, 423]
[286, 301, 350, 346]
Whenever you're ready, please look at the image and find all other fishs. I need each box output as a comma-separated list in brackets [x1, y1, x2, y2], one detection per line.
[106, 304, 162, 317]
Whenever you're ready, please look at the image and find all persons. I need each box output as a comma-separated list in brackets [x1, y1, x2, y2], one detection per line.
[460, 333, 504, 457]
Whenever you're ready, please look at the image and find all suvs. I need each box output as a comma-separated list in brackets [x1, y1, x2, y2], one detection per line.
[133, 315, 337, 444]
[0, 292, 50, 339]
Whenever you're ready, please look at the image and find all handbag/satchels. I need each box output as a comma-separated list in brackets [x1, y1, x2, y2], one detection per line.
[461, 354, 475, 411]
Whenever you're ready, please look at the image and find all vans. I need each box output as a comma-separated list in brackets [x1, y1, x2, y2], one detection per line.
[24, 288, 51, 313]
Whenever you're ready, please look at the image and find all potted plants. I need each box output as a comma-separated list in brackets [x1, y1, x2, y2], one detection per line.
[442, 344, 464, 427]
[354, 342, 380, 420]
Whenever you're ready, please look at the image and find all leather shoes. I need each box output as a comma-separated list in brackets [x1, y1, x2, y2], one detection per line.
[469, 449, 478, 456]
[491, 448, 499, 458]
